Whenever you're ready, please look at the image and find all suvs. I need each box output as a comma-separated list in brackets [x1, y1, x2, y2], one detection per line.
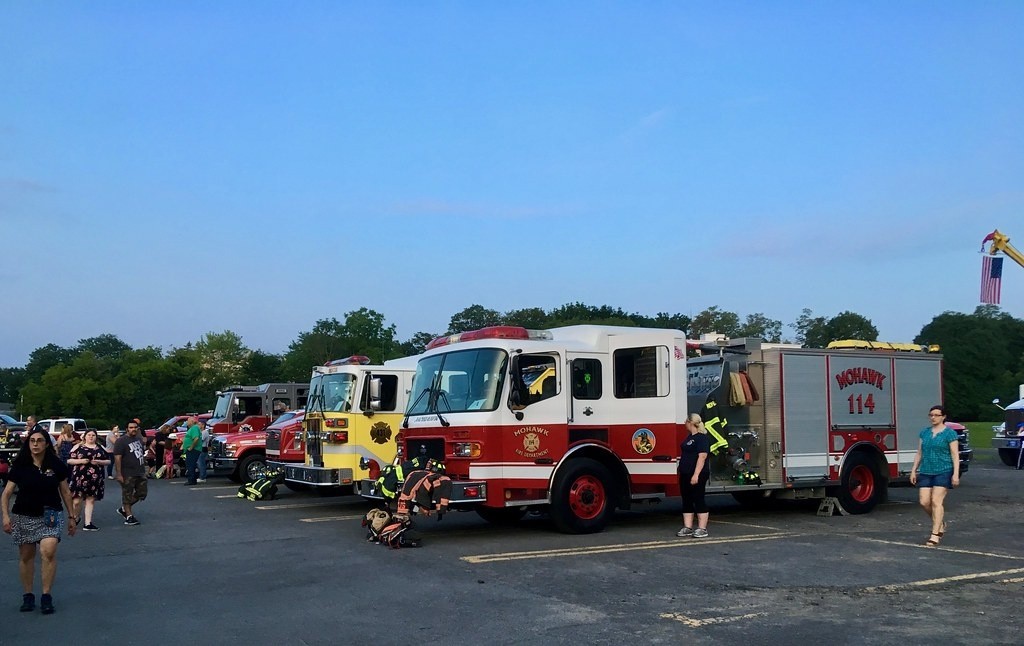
[944, 421, 972, 480]
[0, 414, 28, 432]
[145, 412, 213, 449]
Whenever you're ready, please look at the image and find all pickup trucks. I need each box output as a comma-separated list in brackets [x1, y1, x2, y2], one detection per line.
[0, 448, 20, 483]
[23, 418, 127, 439]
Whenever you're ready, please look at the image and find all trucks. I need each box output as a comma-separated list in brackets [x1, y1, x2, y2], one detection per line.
[992, 385, 1024, 470]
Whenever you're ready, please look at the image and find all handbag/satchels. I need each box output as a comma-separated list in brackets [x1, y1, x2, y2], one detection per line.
[404, 530, 423, 547]
[202, 447, 208, 452]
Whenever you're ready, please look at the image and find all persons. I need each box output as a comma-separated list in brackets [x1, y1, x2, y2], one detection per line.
[910, 405, 960, 546]
[0, 426, 78, 614]
[114, 420, 148, 525]
[67, 428, 112, 532]
[0, 416, 210, 488]
[676, 412, 710, 537]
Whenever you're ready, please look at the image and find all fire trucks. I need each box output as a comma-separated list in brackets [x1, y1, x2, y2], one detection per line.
[362, 324, 944, 534]
[204, 382, 350, 495]
[282, 354, 489, 499]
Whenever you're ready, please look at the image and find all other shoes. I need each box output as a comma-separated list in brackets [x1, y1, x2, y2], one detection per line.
[147, 473, 155, 479]
[184, 482, 196, 485]
[75, 518, 81, 527]
[108, 475, 114, 480]
[82, 522, 101, 531]
[196, 478, 206, 482]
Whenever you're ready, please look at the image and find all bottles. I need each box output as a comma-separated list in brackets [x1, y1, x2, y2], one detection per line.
[737, 468, 744, 484]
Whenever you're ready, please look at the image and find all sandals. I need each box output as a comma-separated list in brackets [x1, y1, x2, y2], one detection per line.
[926, 531, 941, 546]
[939, 520, 945, 539]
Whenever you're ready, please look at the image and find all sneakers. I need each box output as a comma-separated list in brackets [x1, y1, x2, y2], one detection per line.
[40, 594, 53, 614]
[20, 593, 35, 612]
[692, 527, 708, 537]
[676, 526, 694, 536]
[124, 515, 140, 525]
[116, 506, 127, 520]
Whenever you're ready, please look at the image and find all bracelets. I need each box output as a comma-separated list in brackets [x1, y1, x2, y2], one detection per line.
[68, 514, 77, 520]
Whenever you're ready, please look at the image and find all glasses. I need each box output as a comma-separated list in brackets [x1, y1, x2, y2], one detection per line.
[928, 414, 943, 416]
[130, 426, 138, 428]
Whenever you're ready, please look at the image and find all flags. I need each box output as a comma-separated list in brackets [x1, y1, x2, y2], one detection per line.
[980, 256, 1003, 306]
